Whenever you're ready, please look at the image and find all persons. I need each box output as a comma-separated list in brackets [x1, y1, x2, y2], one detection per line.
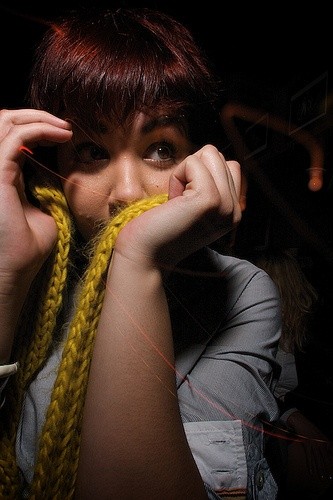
[248, 245, 331, 499]
[2, 12, 319, 500]
